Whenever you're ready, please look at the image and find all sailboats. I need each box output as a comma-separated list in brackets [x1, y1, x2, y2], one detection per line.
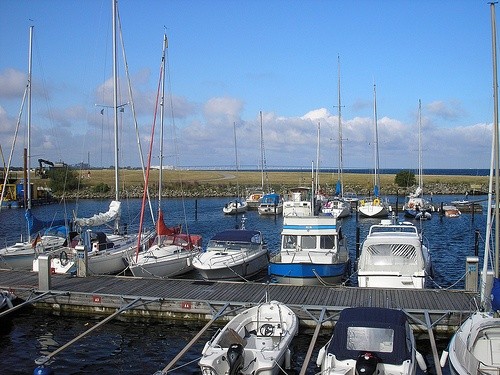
[403, 99, 432, 217]
[222, 121, 249, 213]
[446, 112, 500, 375]
[282, 54, 358, 220]
[32, 0, 158, 276]
[358, 80, 391, 218]
[121, 26, 204, 278]
[245, 111, 266, 208]
[0, 19, 71, 270]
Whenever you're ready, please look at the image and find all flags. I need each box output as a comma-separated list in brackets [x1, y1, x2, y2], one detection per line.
[32, 233, 42, 248]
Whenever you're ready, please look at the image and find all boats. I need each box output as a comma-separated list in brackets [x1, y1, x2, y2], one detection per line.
[191, 213, 272, 282]
[415, 212, 432, 220]
[358, 206, 430, 289]
[444, 209, 462, 218]
[198, 299, 299, 375]
[320, 305, 417, 375]
[0, 146, 57, 209]
[257, 193, 283, 215]
[267, 160, 350, 286]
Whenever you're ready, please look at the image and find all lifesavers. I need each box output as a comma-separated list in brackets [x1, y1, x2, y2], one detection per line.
[374, 199, 380, 206]
